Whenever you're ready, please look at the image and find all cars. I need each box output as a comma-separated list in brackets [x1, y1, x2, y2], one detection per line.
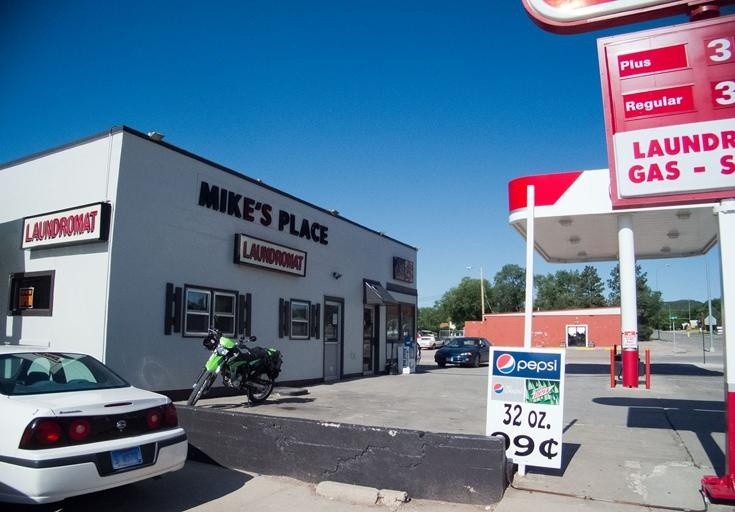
[433, 337, 494, 368]
[1, 345, 190, 505]
[417, 329, 463, 350]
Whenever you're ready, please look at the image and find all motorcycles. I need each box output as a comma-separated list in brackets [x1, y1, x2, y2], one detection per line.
[187, 329, 283, 406]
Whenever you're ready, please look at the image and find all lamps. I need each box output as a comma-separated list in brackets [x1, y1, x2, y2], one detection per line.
[148, 130, 164, 141]
[329, 208, 339, 215]
[333, 272, 342, 279]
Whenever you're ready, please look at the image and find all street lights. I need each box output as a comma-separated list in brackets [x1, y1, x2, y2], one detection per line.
[466, 266, 484, 321]
[657, 262, 670, 340]
